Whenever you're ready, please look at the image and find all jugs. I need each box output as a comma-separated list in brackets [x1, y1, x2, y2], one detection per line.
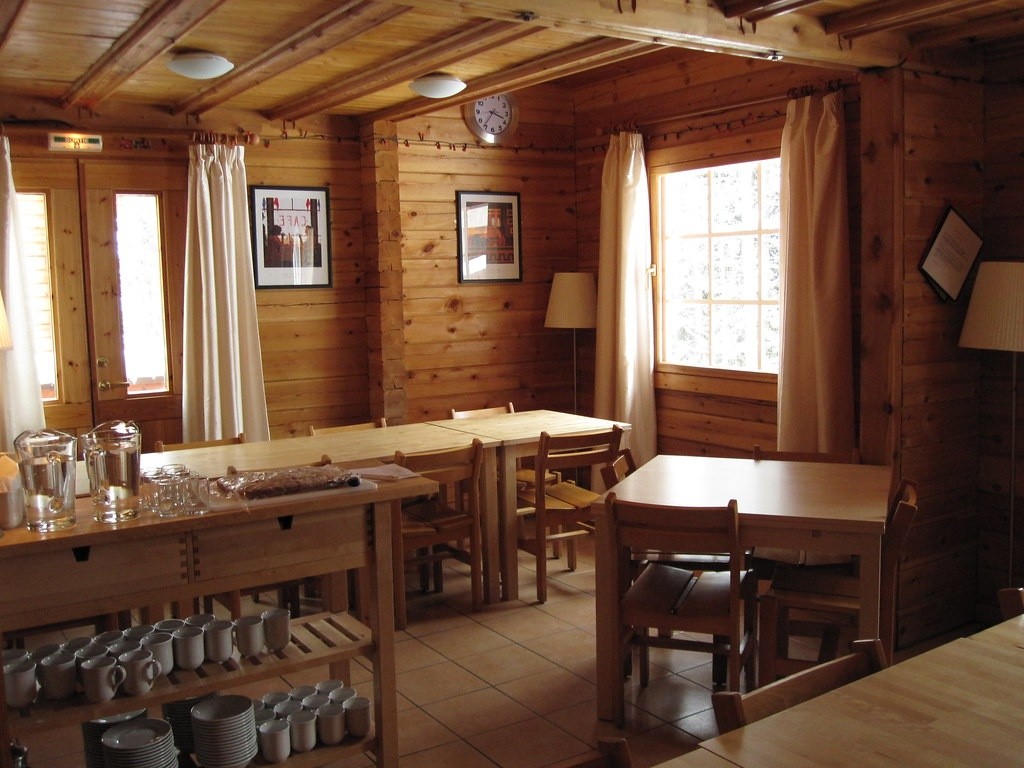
[14, 429, 77, 533]
[80, 420, 142, 524]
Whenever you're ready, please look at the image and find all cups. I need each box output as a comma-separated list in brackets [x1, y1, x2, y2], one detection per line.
[315, 680, 343, 695]
[280, 711, 317, 752]
[144, 464, 211, 518]
[291, 686, 317, 701]
[235, 616, 264, 658]
[302, 694, 331, 710]
[261, 610, 291, 651]
[263, 692, 290, 708]
[0, 490, 23, 529]
[252, 699, 264, 711]
[328, 687, 357, 705]
[257, 721, 291, 763]
[316, 704, 346, 746]
[255, 709, 276, 725]
[274, 700, 303, 718]
[3, 614, 234, 708]
[342, 697, 371, 737]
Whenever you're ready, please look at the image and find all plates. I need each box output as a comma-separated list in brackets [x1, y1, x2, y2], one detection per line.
[82, 691, 258, 768]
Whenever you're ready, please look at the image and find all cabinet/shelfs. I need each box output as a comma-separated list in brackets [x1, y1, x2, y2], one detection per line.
[0, 462, 441, 768]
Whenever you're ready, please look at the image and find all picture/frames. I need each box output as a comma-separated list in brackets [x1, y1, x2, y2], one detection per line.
[250, 184, 333, 288]
[456, 192, 524, 282]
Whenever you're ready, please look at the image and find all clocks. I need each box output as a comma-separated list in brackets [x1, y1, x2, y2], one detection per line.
[464, 92, 520, 144]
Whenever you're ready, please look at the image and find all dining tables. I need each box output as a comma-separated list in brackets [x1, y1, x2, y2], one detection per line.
[589, 454, 892, 719]
[648, 609, 1024, 768]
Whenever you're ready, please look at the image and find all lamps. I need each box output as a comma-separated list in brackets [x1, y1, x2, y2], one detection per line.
[409, 74, 466, 99]
[169, 53, 234, 78]
[958, 262, 1024, 587]
[544, 272, 597, 487]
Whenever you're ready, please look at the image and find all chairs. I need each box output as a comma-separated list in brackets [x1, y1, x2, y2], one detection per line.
[596, 449, 919, 710]
[155, 401, 634, 624]
